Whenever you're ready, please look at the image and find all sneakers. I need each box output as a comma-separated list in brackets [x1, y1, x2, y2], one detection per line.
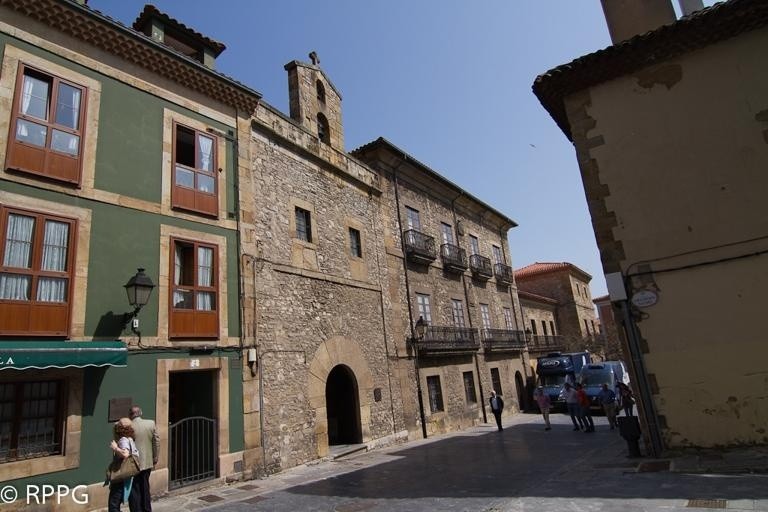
[544, 422, 552, 431]
[572, 424, 595, 433]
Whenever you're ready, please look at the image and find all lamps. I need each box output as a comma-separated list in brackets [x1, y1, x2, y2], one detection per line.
[524, 327, 532, 343]
[123, 265, 156, 317]
[411, 315, 428, 344]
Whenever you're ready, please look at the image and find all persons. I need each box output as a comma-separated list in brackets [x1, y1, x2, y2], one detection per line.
[564, 382, 595, 434]
[106, 405, 160, 512]
[537, 386, 553, 430]
[489, 390, 504, 432]
[598, 383, 635, 431]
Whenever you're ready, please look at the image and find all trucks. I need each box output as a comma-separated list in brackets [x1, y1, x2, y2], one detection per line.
[533, 351, 591, 413]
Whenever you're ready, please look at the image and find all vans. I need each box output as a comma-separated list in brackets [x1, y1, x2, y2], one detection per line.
[568, 359, 628, 417]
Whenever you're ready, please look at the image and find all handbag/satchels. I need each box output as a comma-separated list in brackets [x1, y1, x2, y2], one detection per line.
[105, 437, 142, 481]
